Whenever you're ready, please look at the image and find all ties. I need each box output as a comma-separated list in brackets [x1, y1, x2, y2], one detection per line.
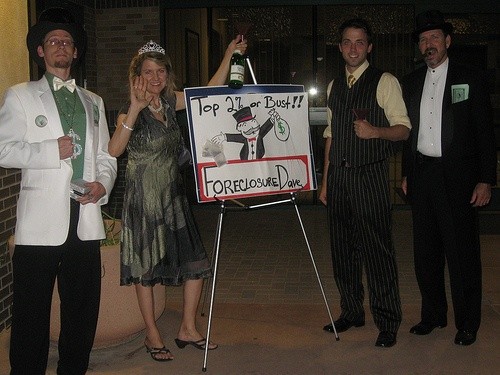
[348, 75, 355, 88]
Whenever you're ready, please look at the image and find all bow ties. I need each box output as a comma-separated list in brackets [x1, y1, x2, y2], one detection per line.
[52, 76, 78, 93]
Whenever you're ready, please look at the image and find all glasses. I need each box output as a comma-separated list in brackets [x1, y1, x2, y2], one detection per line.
[45, 38, 75, 47]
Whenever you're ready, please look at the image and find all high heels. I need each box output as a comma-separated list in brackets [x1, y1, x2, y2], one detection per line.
[174, 337, 218, 351]
[145, 340, 174, 361]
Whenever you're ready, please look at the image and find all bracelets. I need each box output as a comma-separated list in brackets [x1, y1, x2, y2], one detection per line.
[121, 122, 134, 130]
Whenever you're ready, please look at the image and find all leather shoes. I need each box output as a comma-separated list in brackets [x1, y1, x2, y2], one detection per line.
[454, 327, 478, 346]
[323, 318, 366, 333]
[410, 320, 448, 335]
[375, 331, 397, 347]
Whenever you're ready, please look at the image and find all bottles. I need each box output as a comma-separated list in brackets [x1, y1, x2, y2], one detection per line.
[227, 33, 246, 88]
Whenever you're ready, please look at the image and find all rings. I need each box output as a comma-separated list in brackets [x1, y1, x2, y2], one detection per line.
[88, 192, 94, 200]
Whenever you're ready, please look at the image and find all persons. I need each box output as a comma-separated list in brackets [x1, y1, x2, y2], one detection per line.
[0, 8, 116, 375]
[402, 11, 497, 344]
[108, 34, 247, 361]
[319, 16, 412, 345]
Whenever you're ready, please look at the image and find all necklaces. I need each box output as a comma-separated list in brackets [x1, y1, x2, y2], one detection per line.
[147, 97, 163, 113]
[54, 89, 77, 133]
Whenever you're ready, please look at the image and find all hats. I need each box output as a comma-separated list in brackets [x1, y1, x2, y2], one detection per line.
[26, 7, 87, 65]
[410, 10, 454, 42]
